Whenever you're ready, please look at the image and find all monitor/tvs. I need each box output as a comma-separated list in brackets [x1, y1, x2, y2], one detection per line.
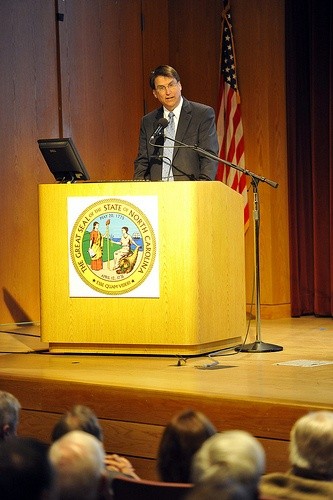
[37, 138, 89, 183]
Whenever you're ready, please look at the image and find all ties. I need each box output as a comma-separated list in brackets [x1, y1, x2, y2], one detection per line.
[162, 112, 175, 182]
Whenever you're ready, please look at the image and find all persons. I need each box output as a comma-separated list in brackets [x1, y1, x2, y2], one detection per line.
[193, 429, 267, 500]
[1, 390, 141, 500]
[271, 410, 332, 494]
[133, 65, 220, 182]
[156, 410, 218, 485]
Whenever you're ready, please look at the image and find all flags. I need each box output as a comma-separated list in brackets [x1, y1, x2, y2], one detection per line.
[215, 6, 250, 237]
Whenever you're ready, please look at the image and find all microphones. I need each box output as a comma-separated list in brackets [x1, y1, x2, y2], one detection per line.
[151, 118, 168, 139]
